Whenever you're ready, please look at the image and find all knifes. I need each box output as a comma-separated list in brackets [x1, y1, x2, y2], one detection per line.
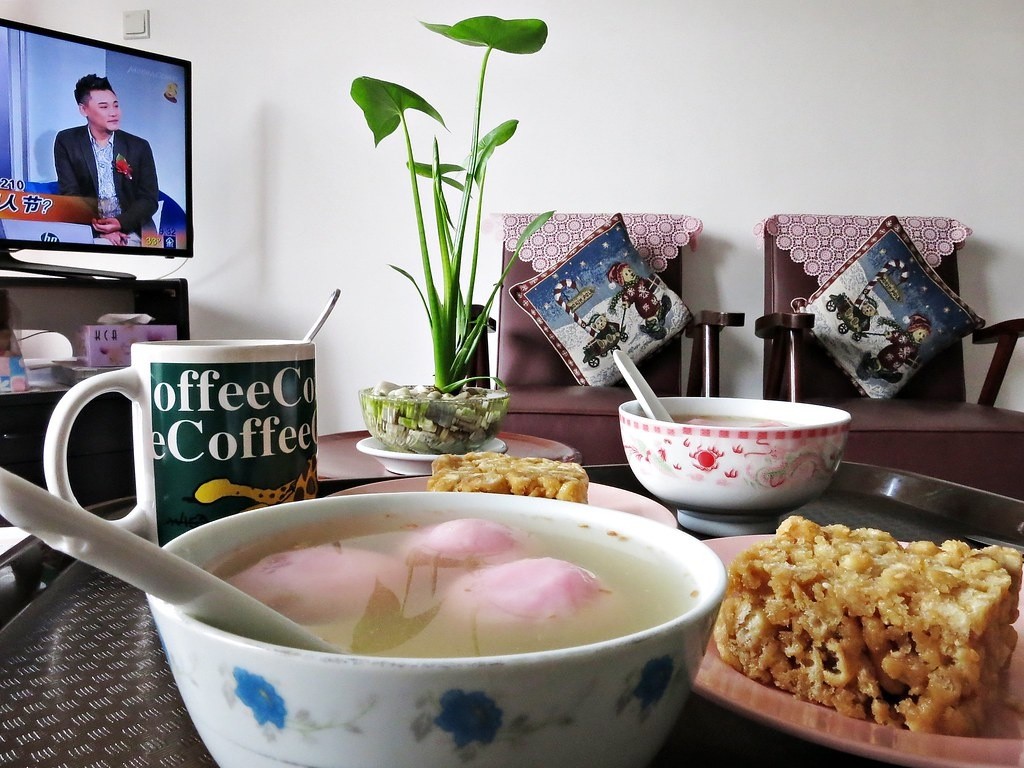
[964, 535, 1024, 556]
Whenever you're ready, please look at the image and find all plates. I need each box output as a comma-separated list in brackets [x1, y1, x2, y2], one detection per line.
[325, 476, 678, 531]
[355, 437, 507, 476]
[678, 534, 1023, 767]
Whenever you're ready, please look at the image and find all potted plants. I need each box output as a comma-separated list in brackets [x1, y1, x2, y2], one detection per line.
[350, 16, 549, 453]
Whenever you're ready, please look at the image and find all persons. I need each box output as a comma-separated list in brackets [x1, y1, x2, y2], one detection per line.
[54, 73, 159, 246]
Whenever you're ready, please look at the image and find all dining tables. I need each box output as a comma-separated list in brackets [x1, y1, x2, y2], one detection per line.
[0, 457, 1024, 768]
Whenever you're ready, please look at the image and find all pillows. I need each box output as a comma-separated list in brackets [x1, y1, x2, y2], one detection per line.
[791, 216, 986, 398]
[509, 212, 693, 388]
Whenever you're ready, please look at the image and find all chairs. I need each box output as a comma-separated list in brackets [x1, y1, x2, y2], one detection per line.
[755, 214, 1024, 502]
[496, 211, 746, 470]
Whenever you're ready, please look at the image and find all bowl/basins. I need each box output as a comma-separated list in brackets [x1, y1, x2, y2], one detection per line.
[618, 397, 851, 537]
[144, 491, 728, 767]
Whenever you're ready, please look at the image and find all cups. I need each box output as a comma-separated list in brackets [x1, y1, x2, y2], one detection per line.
[43, 340, 319, 548]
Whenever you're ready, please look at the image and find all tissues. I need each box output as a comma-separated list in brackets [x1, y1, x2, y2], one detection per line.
[75, 313, 177, 368]
